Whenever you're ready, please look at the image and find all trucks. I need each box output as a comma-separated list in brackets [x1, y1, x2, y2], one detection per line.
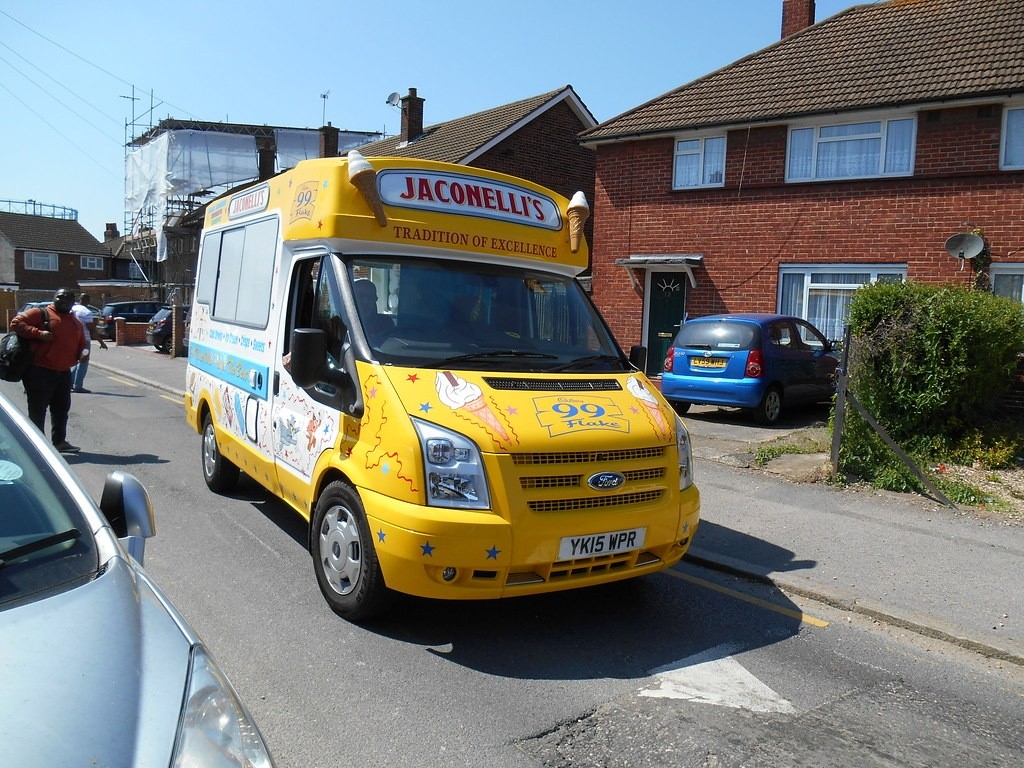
[184, 148, 701, 622]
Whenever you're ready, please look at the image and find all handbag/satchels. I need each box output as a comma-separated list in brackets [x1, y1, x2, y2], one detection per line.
[0, 308, 50, 382]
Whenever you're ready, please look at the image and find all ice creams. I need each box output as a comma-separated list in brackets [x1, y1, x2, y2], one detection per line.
[567, 191, 590, 254]
[347, 150, 387, 228]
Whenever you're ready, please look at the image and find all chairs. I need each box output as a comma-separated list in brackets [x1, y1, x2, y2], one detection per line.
[772, 329, 782, 344]
[332, 279, 395, 340]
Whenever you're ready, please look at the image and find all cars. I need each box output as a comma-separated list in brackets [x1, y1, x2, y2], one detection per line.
[16, 302, 101, 316]
[96, 301, 171, 342]
[0, 392, 277, 768]
[661, 312, 843, 429]
[183, 307, 192, 347]
[145, 305, 191, 355]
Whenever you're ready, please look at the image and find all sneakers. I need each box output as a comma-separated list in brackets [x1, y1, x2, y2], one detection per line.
[54, 441, 81, 452]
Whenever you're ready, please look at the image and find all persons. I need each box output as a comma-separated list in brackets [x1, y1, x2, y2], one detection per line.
[10, 288, 91, 453]
[332, 277, 394, 360]
[70, 291, 109, 392]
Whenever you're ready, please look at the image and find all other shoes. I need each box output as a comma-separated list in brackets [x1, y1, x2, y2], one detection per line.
[74, 388, 91, 393]
